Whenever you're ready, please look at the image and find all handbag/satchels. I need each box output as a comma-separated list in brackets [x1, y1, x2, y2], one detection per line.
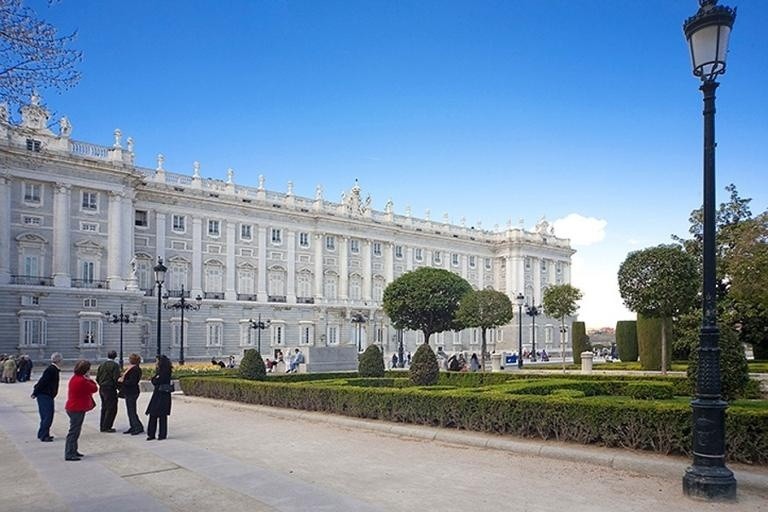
[158, 384, 174, 393]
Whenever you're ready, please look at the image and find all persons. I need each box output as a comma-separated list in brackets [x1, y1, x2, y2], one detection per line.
[95, 351, 121, 432]
[265, 349, 304, 372]
[116, 353, 143, 434]
[145, 355, 172, 441]
[436, 348, 479, 370]
[0, 354, 32, 383]
[392, 352, 412, 368]
[31, 352, 63, 442]
[64, 361, 98, 461]
[211, 354, 236, 369]
[522, 350, 550, 361]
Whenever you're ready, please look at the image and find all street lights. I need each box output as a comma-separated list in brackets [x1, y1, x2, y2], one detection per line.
[153, 255, 168, 361]
[683, 1, 740, 502]
[160, 281, 203, 369]
[517, 292, 524, 367]
[248, 312, 272, 356]
[526, 296, 543, 361]
[350, 313, 366, 351]
[105, 304, 138, 370]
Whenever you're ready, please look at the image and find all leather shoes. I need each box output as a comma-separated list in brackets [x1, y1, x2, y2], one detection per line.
[102, 429, 115, 432]
[147, 436, 155, 440]
[67, 453, 83, 460]
[124, 428, 143, 434]
